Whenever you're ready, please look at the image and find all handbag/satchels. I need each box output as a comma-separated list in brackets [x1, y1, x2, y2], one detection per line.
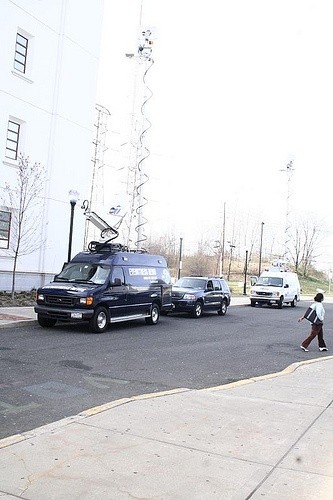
[304, 306, 321, 325]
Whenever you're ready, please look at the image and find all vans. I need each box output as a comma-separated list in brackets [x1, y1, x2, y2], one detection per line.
[250, 271, 301, 309]
[171, 276, 231, 318]
[34, 211, 175, 334]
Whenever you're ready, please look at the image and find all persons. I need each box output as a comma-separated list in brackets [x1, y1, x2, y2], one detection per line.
[298, 293, 328, 352]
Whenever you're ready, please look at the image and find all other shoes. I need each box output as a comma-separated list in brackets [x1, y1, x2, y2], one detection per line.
[318, 347, 329, 352]
[300, 345, 309, 351]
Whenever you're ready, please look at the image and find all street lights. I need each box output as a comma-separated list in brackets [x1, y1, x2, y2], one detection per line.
[178, 233, 185, 279]
[244, 245, 248, 295]
[67, 189, 79, 262]
